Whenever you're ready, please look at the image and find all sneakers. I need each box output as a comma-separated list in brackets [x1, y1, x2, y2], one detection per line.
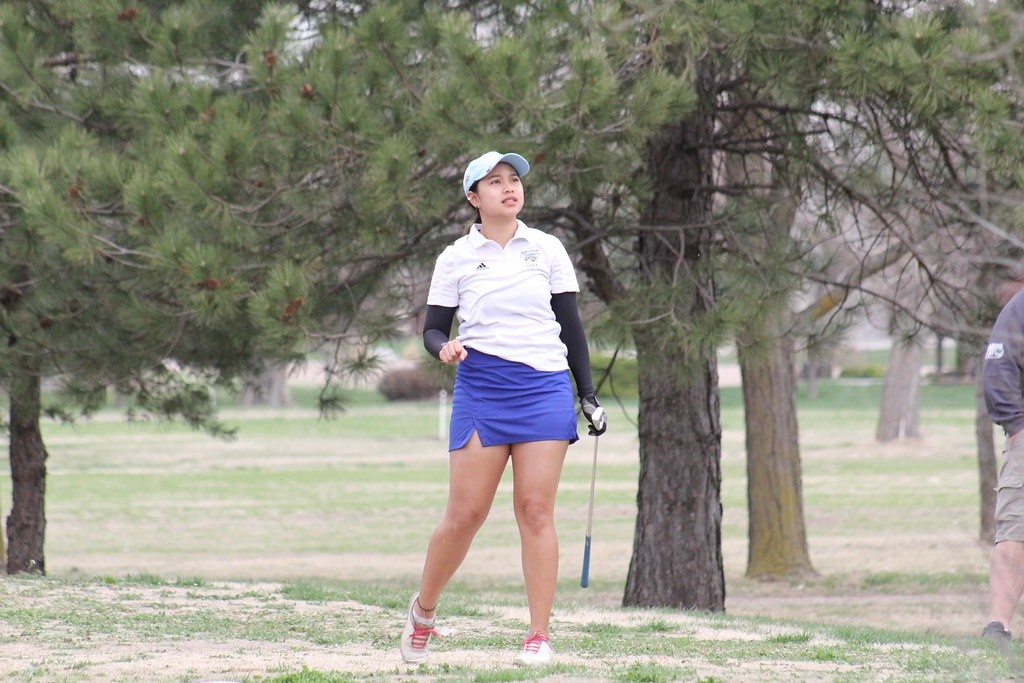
[513, 633, 552, 666]
[981, 621, 1012, 641]
[399, 591, 436, 664]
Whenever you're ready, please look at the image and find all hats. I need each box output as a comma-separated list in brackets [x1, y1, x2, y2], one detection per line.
[463, 151, 530, 195]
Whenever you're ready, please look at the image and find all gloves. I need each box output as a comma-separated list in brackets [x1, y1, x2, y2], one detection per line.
[579, 396, 606, 436]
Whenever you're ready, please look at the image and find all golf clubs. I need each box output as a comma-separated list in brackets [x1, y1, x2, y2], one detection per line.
[580, 407, 607, 590]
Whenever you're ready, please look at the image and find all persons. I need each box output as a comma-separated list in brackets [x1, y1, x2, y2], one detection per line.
[401, 151, 608, 668]
[982, 283, 1023, 640]
